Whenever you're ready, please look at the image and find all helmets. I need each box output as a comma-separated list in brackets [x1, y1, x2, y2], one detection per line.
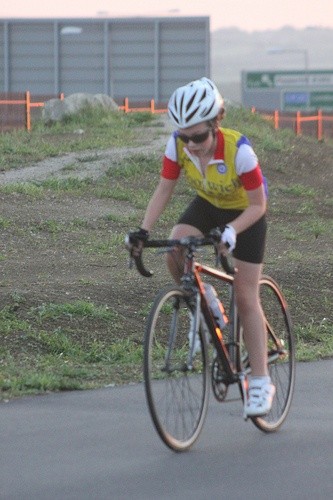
[168, 77, 223, 130]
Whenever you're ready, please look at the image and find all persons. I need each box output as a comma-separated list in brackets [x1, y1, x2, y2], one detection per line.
[125, 77, 277, 416]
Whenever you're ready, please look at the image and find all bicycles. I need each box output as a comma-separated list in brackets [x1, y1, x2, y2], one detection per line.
[124, 226, 296, 453]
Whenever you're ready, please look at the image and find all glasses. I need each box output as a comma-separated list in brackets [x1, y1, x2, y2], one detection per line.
[181, 126, 211, 143]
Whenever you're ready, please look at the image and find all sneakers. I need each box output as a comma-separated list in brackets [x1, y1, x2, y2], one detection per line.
[188, 300, 228, 349]
[245, 376, 276, 416]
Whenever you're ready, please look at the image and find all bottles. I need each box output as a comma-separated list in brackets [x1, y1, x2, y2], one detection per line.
[202, 283, 229, 330]
